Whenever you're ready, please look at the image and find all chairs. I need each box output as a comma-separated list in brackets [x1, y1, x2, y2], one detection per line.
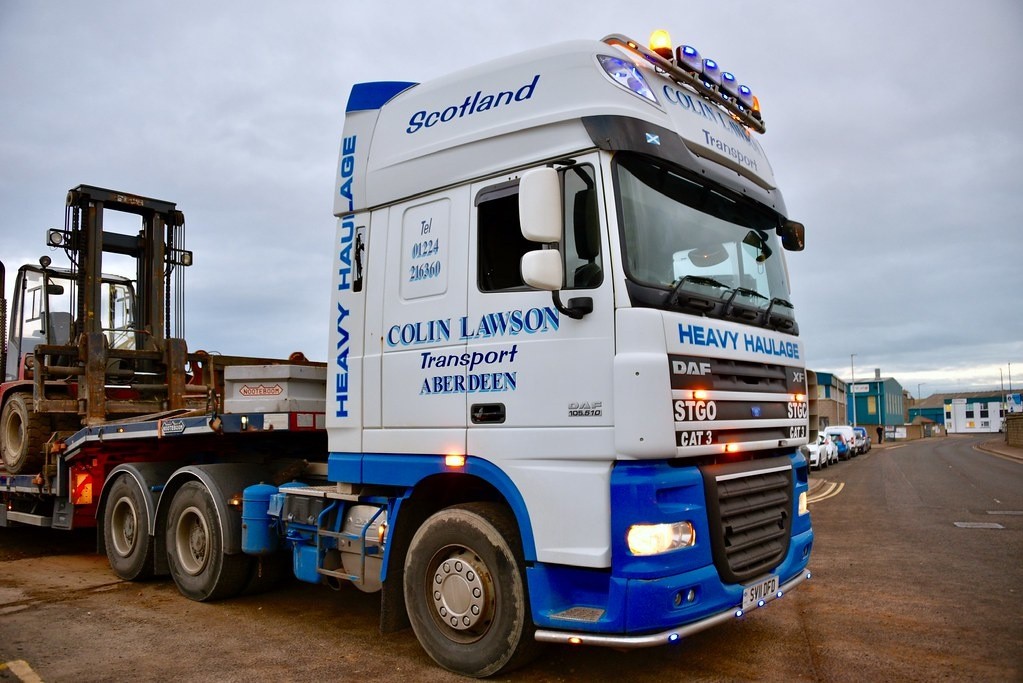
[46, 312, 73, 346]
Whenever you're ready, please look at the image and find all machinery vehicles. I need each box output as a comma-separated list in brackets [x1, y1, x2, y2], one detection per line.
[0, 179, 228, 475]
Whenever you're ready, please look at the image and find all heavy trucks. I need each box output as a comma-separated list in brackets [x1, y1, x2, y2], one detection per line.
[0, 28, 816, 681]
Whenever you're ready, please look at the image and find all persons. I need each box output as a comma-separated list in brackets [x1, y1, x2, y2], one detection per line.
[876, 425, 883, 444]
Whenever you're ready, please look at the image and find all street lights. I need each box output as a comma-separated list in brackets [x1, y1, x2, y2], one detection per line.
[918, 382, 923, 416]
[1008, 363, 1012, 393]
[850, 352, 856, 427]
[999, 368, 1006, 417]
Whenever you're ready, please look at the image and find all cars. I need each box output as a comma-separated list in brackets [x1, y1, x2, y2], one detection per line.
[807, 426, 872, 470]
